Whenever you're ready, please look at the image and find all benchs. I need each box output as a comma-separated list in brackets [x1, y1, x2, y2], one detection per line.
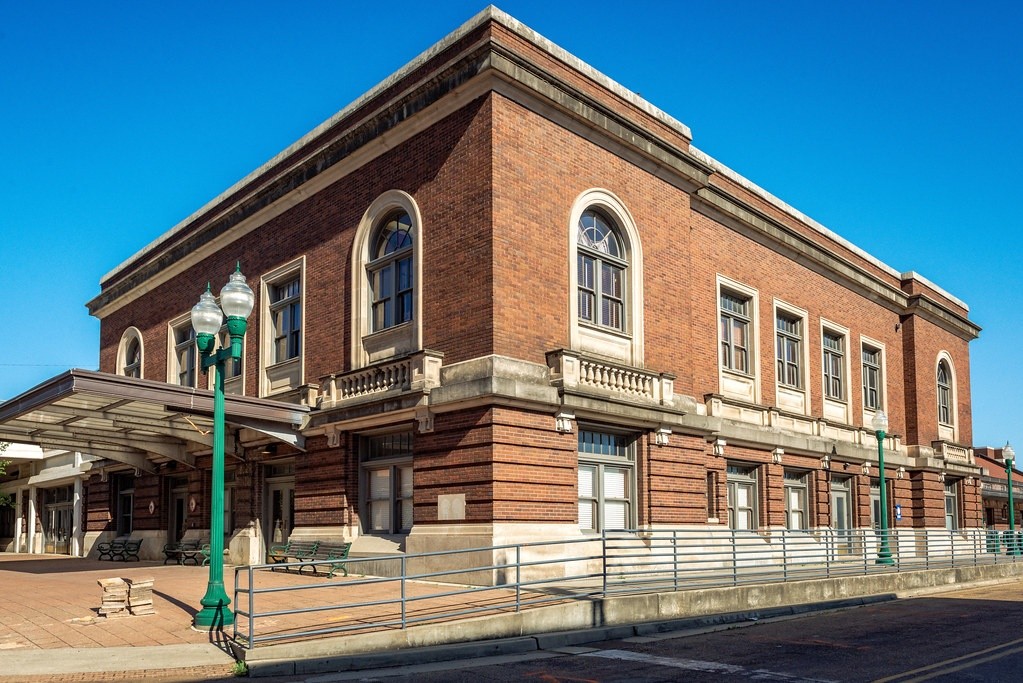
[269, 540, 352, 578]
[96, 538, 144, 562]
[162, 539, 210, 566]
